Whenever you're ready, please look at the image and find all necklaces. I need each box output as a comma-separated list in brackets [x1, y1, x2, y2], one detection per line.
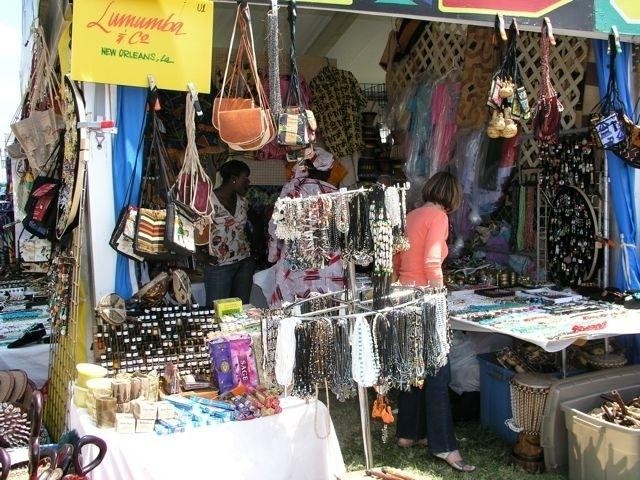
[272, 283, 454, 442]
[273, 179, 410, 279]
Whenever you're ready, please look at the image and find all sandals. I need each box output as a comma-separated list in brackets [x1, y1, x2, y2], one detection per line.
[432, 450, 476, 472]
[396, 438, 428, 448]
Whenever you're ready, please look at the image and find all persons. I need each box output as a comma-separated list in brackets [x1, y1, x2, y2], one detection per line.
[390, 172, 477, 473]
[205, 159, 255, 309]
[270, 147, 346, 309]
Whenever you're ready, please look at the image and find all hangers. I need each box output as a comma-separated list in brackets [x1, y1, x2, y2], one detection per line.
[491, 13, 508, 44]
[537, 17, 556, 45]
[512, 18, 520, 36]
[431, 56, 463, 89]
[606, 25, 622, 55]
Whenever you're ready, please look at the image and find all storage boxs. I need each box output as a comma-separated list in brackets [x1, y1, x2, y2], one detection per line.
[558, 385, 640, 480]
[475, 352, 594, 444]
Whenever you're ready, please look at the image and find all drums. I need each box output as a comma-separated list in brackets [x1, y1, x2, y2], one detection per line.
[585, 354, 626, 374]
[504, 372, 556, 474]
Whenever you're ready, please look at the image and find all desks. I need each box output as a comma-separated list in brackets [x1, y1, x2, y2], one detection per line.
[65, 380, 347, 480]
[0, 304, 57, 390]
[448, 283, 640, 380]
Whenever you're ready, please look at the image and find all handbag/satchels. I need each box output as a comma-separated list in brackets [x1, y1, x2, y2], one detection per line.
[283, 144, 316, 163]
[134, 208, 178, 257]
[284, 105, 318, 149]
[216, 108, 269, 152]
[593, 112, 625, 149]
[276, 113, 305, 146]
[164, 199, 197, 257]
[212, 97, 253, 130]
[264, 109, 277, 144]
[613, 114, 640, 169]
[108, 205, 145, 263]
[21, 175, 58, 239]
[175, 170, 217, 214]
